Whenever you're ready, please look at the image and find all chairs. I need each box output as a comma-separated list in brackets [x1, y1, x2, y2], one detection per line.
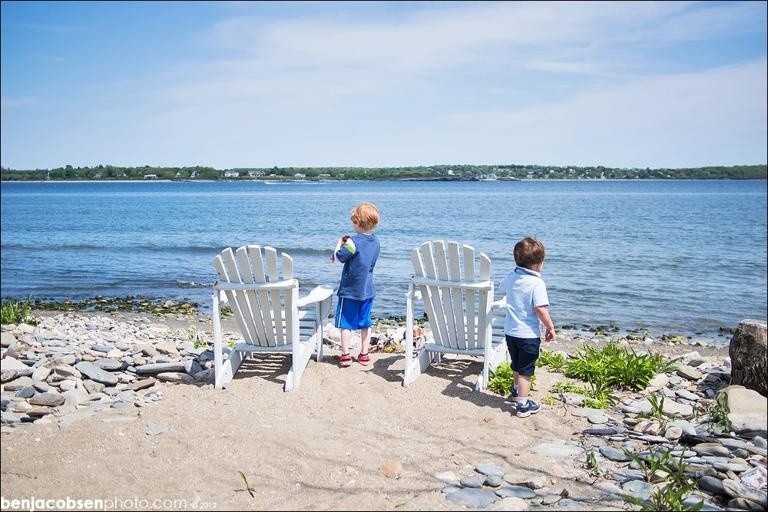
[211, 244, 338, 394]
[401, 240, 510, 394]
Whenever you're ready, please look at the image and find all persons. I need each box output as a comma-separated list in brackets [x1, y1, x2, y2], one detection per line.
[496, 236, 556, 418]
[333, 202, 381, 367]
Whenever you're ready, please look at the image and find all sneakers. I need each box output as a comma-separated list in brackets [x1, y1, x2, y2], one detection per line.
[339, 352, 353, 367]
[356, 352, 371, 365]
[511, 385, 520, 398]
[514, 398, 542, 418]
